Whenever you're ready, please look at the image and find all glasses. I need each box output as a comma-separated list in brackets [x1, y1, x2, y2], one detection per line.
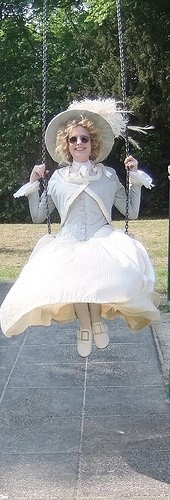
[67, 136, 90, 144]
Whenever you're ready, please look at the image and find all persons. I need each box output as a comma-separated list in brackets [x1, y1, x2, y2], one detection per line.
[1, 98, 160, 358]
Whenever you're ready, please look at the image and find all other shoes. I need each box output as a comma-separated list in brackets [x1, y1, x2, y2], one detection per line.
[92, 321, 109, 349]
[77, 320, 92, 358]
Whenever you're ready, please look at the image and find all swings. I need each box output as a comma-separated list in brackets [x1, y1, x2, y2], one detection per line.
[41, 0, 130, 235]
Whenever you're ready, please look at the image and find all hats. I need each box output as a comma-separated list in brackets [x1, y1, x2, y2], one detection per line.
[45, 98, 129, 166]
